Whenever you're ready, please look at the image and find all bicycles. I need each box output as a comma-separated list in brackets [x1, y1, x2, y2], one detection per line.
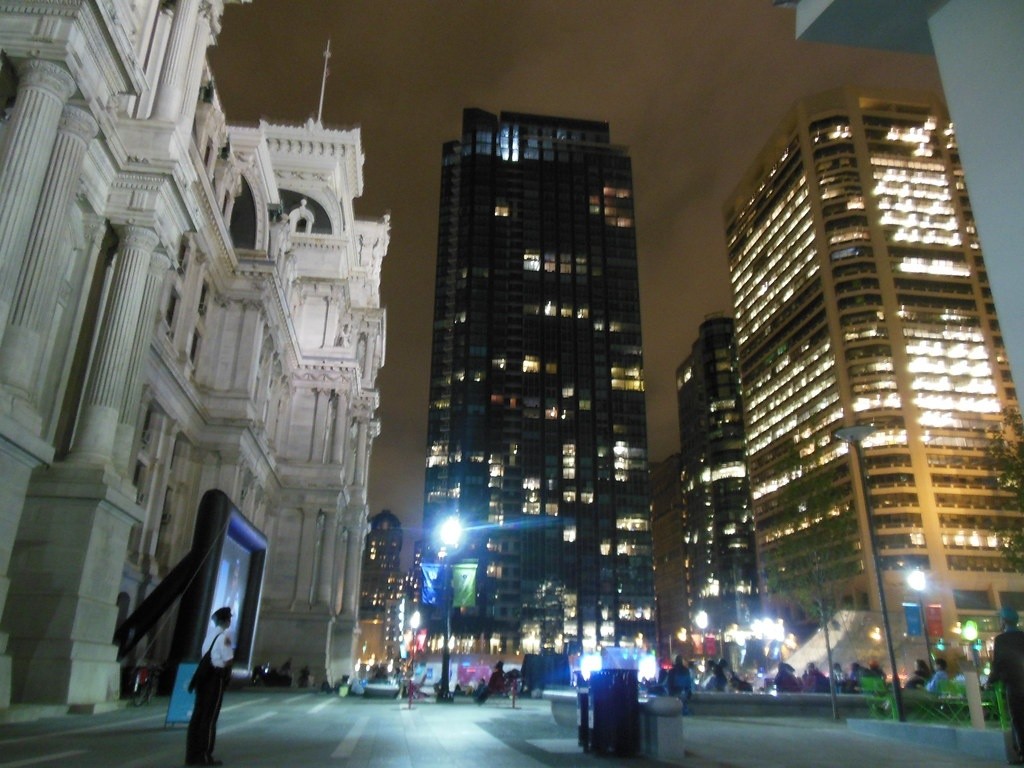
[128, 658, 164, 706]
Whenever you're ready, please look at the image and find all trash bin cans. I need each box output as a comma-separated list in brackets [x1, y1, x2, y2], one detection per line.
[575, 668, 640, 760]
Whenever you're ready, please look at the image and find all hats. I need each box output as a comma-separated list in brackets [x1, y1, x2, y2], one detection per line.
[211, 607, 233, 623]
[999, 606, 1018, 621]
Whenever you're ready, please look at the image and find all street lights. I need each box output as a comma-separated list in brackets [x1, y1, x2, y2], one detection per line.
[903, 566, 934, 672]
[434, 512, 464, 702]
[834, 425, 907, 722]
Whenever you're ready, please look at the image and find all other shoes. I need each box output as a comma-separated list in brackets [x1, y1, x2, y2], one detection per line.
[1006, 755, 1024, 765]
[207, 759, 223, 767]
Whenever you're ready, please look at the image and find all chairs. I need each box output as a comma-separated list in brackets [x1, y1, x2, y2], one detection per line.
[859, 675, 1010, 727]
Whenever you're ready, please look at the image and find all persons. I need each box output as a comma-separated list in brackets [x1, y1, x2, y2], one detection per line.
[473, 661, 506, 706]
[985, 607, 1024, 765]
[185, 607, 233, 768]
[641, 652, 950, 715]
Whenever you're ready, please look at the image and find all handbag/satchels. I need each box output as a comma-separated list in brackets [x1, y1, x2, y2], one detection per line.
[188, 652, 213, 694]
